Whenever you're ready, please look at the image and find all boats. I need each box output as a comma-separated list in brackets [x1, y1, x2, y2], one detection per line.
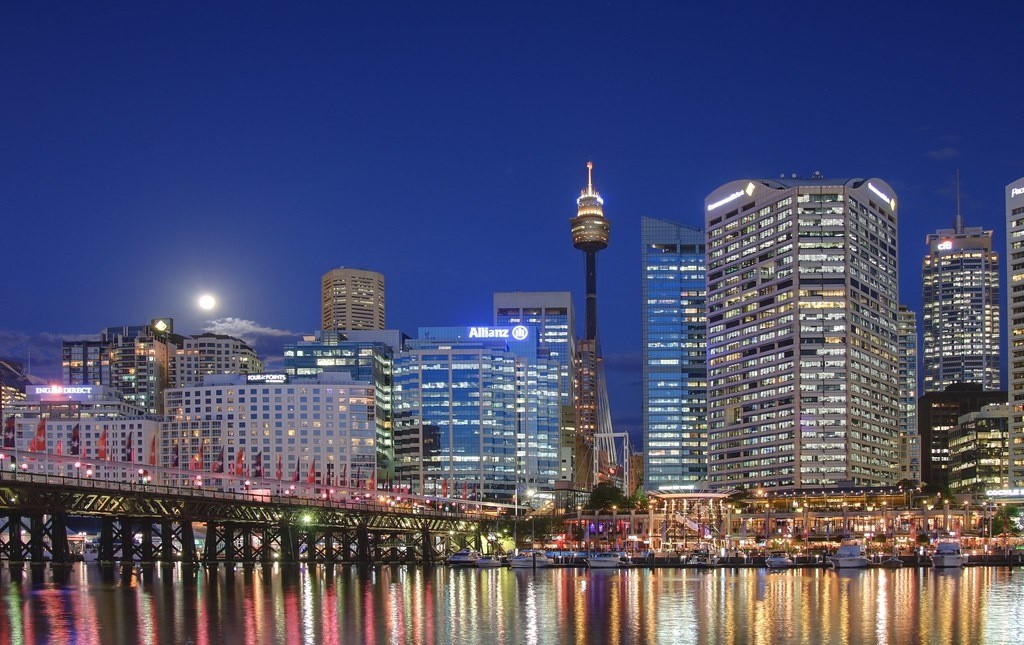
[445, 546, 503, 568]
[583, 551, 622, 568]
[831, 545, 871, 567]
[505, 550, 554, 569]
[765, 550, 792, 569]
[930, 542, 965, 568]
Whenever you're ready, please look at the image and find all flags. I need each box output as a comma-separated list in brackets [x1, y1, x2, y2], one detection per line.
[585, 517, 963, 544]
[4, 414, 478, 500]
